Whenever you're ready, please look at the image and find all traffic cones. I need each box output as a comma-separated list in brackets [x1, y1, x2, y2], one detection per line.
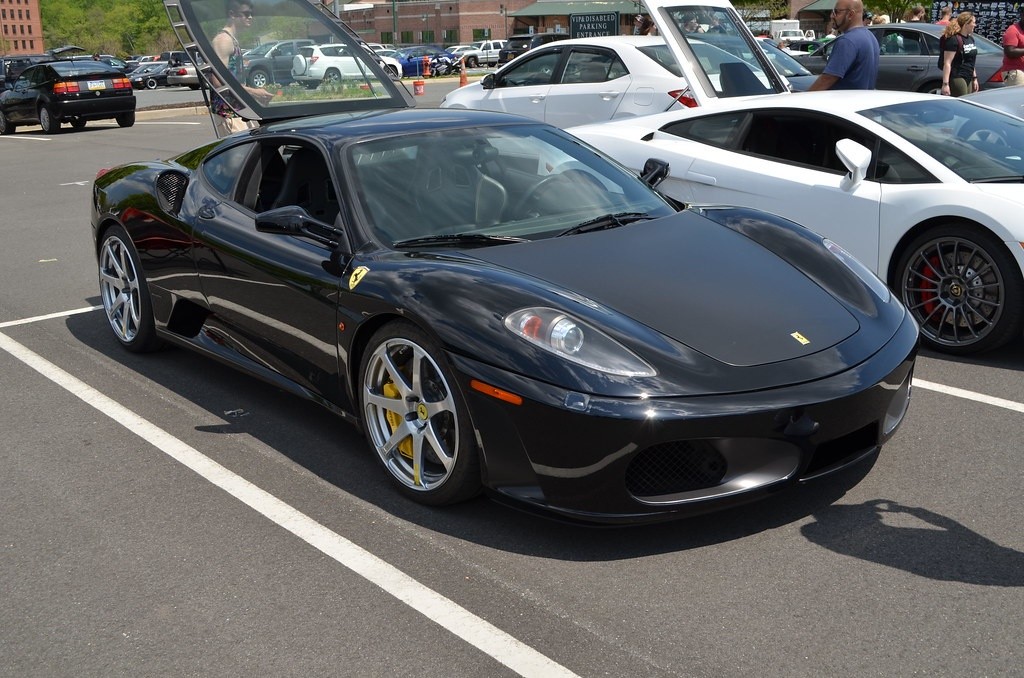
[460, 59, 469, 87]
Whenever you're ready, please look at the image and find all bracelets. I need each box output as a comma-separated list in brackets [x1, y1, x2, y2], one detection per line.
[973, 77, 978, 78]
[943, 82, 949, 85]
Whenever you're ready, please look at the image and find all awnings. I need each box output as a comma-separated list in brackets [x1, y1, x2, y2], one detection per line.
[506, 0, 649, 31]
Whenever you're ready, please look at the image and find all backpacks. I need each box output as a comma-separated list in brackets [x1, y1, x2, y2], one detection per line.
[938, 32, 962, 70]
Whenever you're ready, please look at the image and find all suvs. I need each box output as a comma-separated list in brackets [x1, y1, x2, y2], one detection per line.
[454, 40, 509, 68]
[0, 46, 88, 94]
[290, 42, 403, 89]
[497, 33, 582, 79]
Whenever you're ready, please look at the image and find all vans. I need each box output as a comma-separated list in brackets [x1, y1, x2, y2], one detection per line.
[237, 39, 315, 89]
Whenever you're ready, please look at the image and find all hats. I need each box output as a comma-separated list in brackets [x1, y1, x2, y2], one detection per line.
[862, 12, 873, 20]
[1018, 3, 1024, 15]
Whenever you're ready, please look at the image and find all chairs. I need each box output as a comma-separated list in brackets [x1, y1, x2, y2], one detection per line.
[580, 62, 607, 81]
[271, 144, 406, 245]
[405, 142, 508, 234]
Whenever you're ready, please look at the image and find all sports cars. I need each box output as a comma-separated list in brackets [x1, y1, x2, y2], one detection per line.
[92, 0, 919, 524]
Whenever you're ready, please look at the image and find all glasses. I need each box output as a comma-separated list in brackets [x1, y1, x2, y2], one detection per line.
[238, 11, 254, 17]
[833, 8, 851, 16]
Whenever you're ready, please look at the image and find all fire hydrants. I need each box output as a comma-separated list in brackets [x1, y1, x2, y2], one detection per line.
[422, 56, 431, 79]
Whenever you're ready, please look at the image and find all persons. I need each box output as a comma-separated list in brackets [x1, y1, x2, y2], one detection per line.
[825, 10, 886, 39]
[1001, 7, 1024, 86]
[935, 7, 952, 25]
[909, 6, 925, 23]
[633, 13, 726, 37]
[208, 0, 275, 138]
[940, 12, 979, 97]
[806, 0, 880, 93]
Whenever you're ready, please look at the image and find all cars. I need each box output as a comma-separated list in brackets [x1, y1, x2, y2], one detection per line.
[687, 33, 827, 92]
[361, 43, 460, 76]
[437, 35, 793, 130]
[0, 59, 137, 136]
[537, 0, 1024, 356]
[769, 18, 834, 52]
[88, 51, 211, 90]
[791, 23, 1010, 97]
[439, 46, 480, 56]
[952, 85, 1024, 152]
